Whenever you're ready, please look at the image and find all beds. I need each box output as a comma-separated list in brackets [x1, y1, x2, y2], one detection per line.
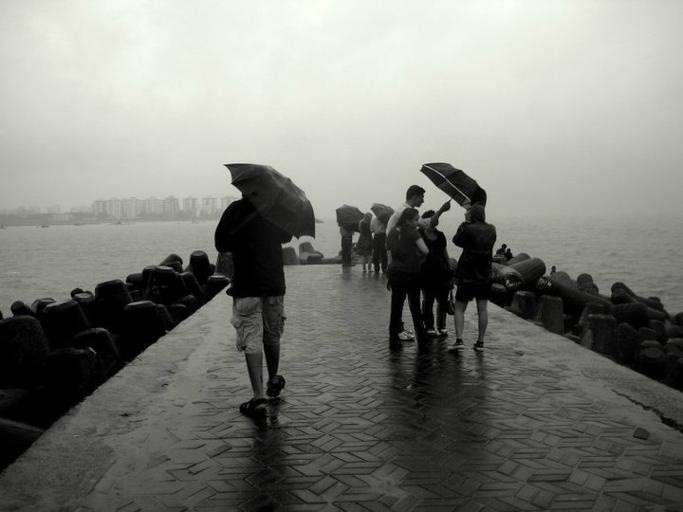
[222, 163, 315, 241]
[336, 202, 395, 232]
[420, 163, 487, 209]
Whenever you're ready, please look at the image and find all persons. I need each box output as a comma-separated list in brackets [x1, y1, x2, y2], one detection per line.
[215, 191, 286, 416]
[386, 185, 496, 352]
[340, 213, 388, 272]
[497, 244, 513, 259]
[550, 265, 556, 275]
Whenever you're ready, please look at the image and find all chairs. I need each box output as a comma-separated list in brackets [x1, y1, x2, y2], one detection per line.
[389, 326, 484, 351]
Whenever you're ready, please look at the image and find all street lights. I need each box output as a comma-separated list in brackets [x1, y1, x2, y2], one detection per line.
[216, 252, 233, 278]
[447, 288, 455, 315]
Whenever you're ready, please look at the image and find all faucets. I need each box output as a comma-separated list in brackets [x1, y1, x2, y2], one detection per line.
[239, 397, 268, 423]
[267, 375, 286, 396]
